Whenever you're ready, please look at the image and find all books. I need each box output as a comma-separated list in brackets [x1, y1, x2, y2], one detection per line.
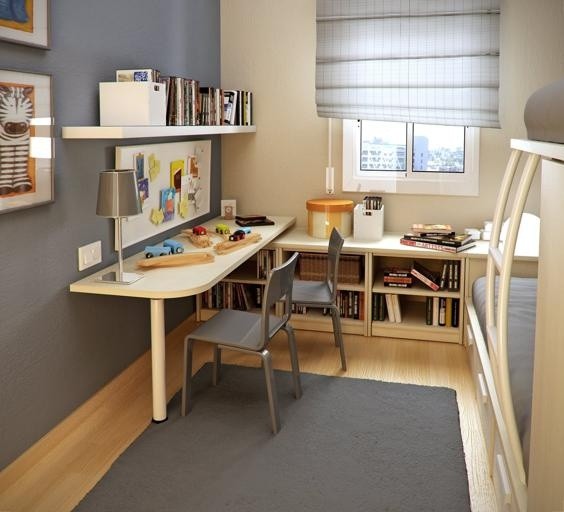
[117, 69, 252, 125]
[235, 215, 275, 226]
[298, 252, 362, 285]
[322, 291, 363, 320]
[201, 282, 262, 312]
[254, 250, 275, 280]
[291, 304, 308, 314]
[363, 195, 382, 215]
[372, 221, 495, 328]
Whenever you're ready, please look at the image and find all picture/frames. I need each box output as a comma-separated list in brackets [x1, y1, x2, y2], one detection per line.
[0, 0, 52, 51]
[0, 67, 55, 216]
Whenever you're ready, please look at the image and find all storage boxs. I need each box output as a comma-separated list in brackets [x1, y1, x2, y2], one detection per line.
[306, 200, 354, 239]
[353, 204, 384, 241]
[97, 81, 167, 127]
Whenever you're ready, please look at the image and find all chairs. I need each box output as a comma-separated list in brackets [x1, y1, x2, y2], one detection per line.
[181, 252, 303, 436]
[279, 227, 348, 372]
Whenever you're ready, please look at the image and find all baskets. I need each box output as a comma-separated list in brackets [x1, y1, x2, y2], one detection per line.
[300, 253, 360, 284]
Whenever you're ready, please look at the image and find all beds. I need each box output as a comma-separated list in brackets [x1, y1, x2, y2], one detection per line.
[461, 78, 564, 511]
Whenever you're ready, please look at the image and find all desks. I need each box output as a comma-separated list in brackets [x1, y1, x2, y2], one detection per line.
[70, 215, 298, 424]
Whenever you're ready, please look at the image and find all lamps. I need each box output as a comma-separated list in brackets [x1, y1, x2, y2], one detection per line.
[96, 169, 143, 285]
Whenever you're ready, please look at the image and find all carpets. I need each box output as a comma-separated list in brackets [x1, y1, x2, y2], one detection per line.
[67, 361, 472, 511]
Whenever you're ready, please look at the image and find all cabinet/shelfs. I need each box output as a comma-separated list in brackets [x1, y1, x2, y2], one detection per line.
[279, 226, 367, 337]
[465, 253, 538, 347]
[369, 233, 466, 345]
[195, 238, 278, 324]
